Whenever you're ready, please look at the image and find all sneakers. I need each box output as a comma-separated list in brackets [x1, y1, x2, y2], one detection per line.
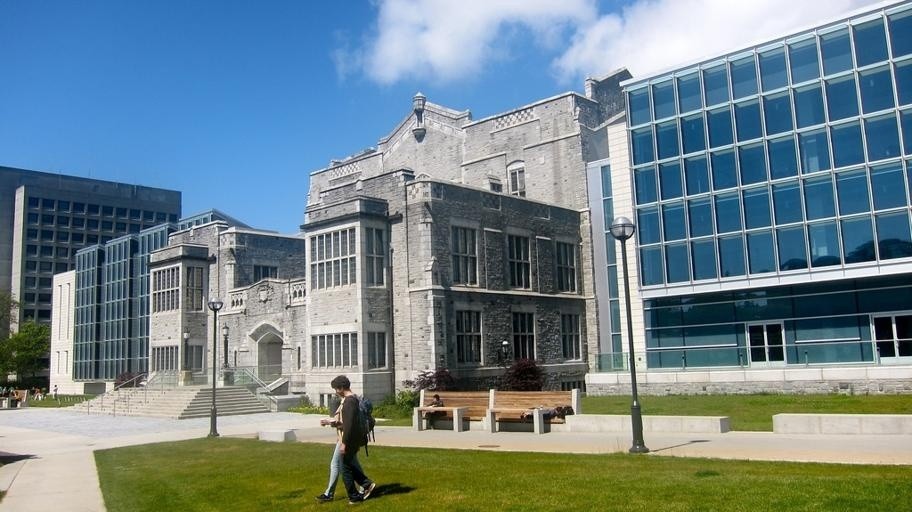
[347, 482, 376, 505]
[315, 494, 333, 501]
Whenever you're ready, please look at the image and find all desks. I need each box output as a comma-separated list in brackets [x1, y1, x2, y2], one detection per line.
[1, 396, 16, 409]
[486, 408, 549, 433]
[412, 406, 467, 432]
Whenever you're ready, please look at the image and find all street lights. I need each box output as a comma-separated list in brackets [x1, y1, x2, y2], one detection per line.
[207, 297, 223, 437]
[222, 321, 230, 368]
[183, 328, 191, 370]
[608, 217, 650, 453]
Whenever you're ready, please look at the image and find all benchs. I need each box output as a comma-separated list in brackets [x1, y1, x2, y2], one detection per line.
[10, 390, 29, 407]
[419, 387, 580, 432]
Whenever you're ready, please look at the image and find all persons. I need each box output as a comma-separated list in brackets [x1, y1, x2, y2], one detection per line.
[315, 388, 365, 501]
[2, 384, 59, 401]
[520, 404, 572, 420]
[331, 375, 376, 504]
[422, 394, 447, 428]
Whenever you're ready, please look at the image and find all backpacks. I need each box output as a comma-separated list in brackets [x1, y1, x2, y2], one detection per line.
[344, 395, 376, 457]
